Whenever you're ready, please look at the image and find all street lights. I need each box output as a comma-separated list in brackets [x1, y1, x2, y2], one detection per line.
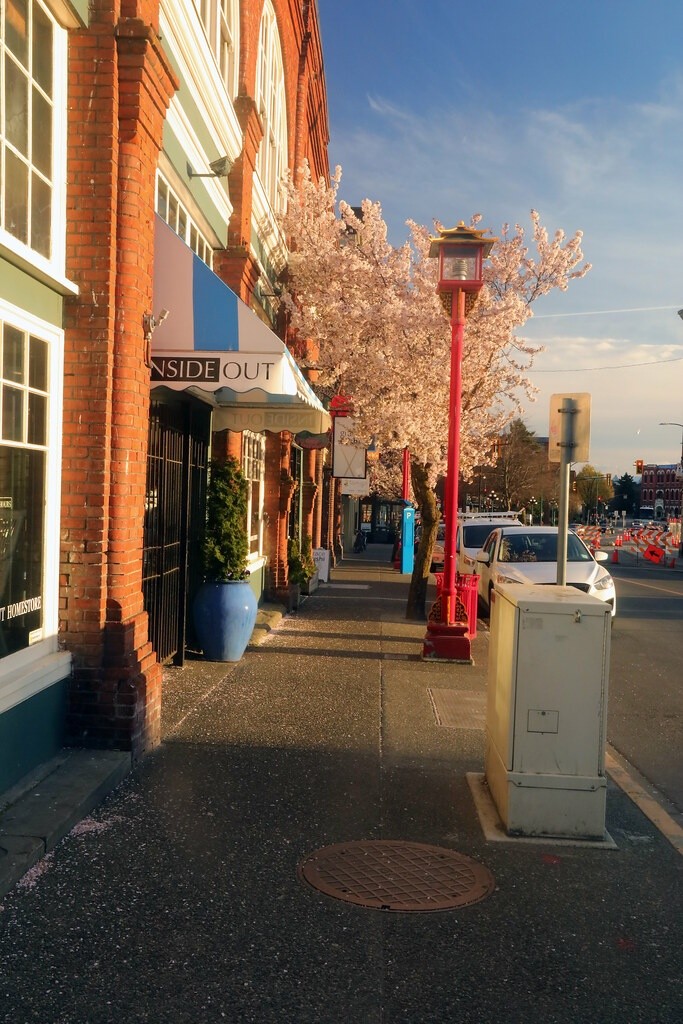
[659, 422, 683, 556]
[487, 489, 500, 516]
[425, 224, 498, 665]
[527, 495, 538, 524]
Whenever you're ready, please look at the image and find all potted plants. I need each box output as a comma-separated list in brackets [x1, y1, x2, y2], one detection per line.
[300, 535, 320, 596]
[191, 450, 257, 661]
[287, 538, 301, 606]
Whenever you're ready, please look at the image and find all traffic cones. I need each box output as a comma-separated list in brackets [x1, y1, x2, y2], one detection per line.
[668, 558, 676, 567]
[610, 543, 621, 564]
[589, 542, 595, 556]
[623, 530, 631, 541]
[614, 534, 623, 546]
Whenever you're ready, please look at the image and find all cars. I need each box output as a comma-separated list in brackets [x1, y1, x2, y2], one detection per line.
[455, 516, 526, 586]
[473, 525, 617, 629]
[414, 526, 422, 552]
[429, 524, 447, 571]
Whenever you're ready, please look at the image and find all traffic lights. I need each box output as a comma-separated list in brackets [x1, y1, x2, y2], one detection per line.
[636, 459, 644, 474]
[598, 495, 601, 502]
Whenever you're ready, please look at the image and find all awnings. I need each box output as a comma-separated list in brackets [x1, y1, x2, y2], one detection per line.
[150, 212, 333, 435]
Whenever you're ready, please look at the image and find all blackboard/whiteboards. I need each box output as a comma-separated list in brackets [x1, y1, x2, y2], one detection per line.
[312, 549, 330, 580]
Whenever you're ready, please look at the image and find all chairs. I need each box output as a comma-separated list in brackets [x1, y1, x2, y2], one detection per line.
[541, 537, 556, 560]
[506, 537, 526, 560]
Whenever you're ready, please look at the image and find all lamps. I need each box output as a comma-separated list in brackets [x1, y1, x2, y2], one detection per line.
[190, 156, 235, 178]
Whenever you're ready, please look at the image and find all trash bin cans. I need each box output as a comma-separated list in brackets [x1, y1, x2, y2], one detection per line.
[434, 573, 479, 639]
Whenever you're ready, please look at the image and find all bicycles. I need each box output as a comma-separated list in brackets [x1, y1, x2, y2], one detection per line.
[353, 526, 370, 553]
